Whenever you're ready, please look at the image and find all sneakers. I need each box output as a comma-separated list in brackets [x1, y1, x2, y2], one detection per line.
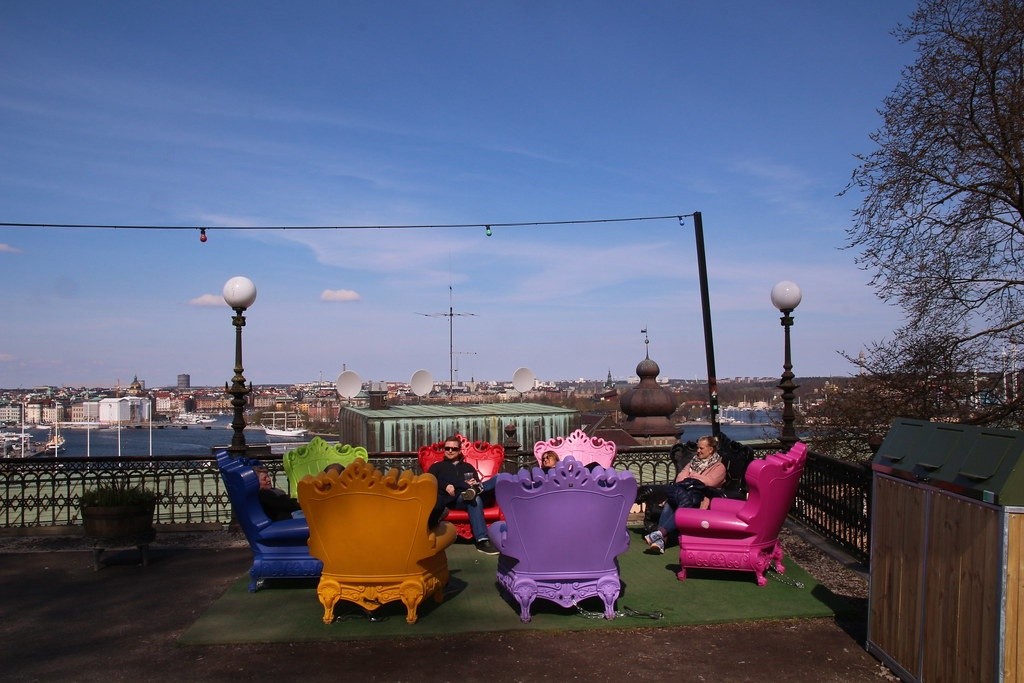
[477, 541, 500, 556]
[461, 488, 477, 501]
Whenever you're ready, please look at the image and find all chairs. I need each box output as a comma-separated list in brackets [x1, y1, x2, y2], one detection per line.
[675, 441, 808, 587]
[297, 458, 456, 625]
[282, 436, 368, 502]
[634, 432, 754, 547]
[418, 433, 506, 539]
[215, 448, 324, 594]
[533, 429, 616, 469]
[486, 451, 637, 624]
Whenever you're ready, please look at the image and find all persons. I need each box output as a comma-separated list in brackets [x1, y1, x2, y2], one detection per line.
[541, 451, 615, 487]
[426, 436, 501, 554]
[645, 437, 727, 554]
[252, 466, 302, 521]
[323, 463, 449, 529]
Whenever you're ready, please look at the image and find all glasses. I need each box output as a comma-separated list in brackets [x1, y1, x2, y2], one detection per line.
[444, 447, 459, 451]
[542, 455, 551, 462]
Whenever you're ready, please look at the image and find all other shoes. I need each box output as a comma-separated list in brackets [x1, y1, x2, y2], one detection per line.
[645, 530, 663, 545]
[651, 539, 664, 554]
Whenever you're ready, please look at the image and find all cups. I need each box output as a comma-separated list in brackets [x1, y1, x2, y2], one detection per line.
[464, 472, 474, 488]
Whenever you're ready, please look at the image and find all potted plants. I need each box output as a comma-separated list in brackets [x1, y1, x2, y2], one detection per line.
[76, 481, 158, 571]
